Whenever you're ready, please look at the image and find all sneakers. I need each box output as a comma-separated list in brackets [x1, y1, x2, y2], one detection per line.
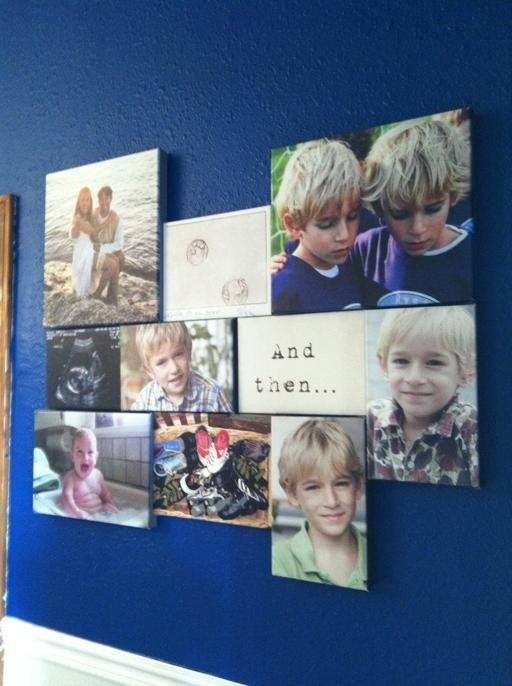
[152, 426, 270, 521]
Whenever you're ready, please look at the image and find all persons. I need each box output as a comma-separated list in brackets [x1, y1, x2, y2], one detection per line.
[366, 307, 478, 488]
[66, 186, 124, 304]
[273, 420, 366, 589]
[58, 428, 120, 519]
[271, 117, 472, 314]
[130, 322, 232, 412]
